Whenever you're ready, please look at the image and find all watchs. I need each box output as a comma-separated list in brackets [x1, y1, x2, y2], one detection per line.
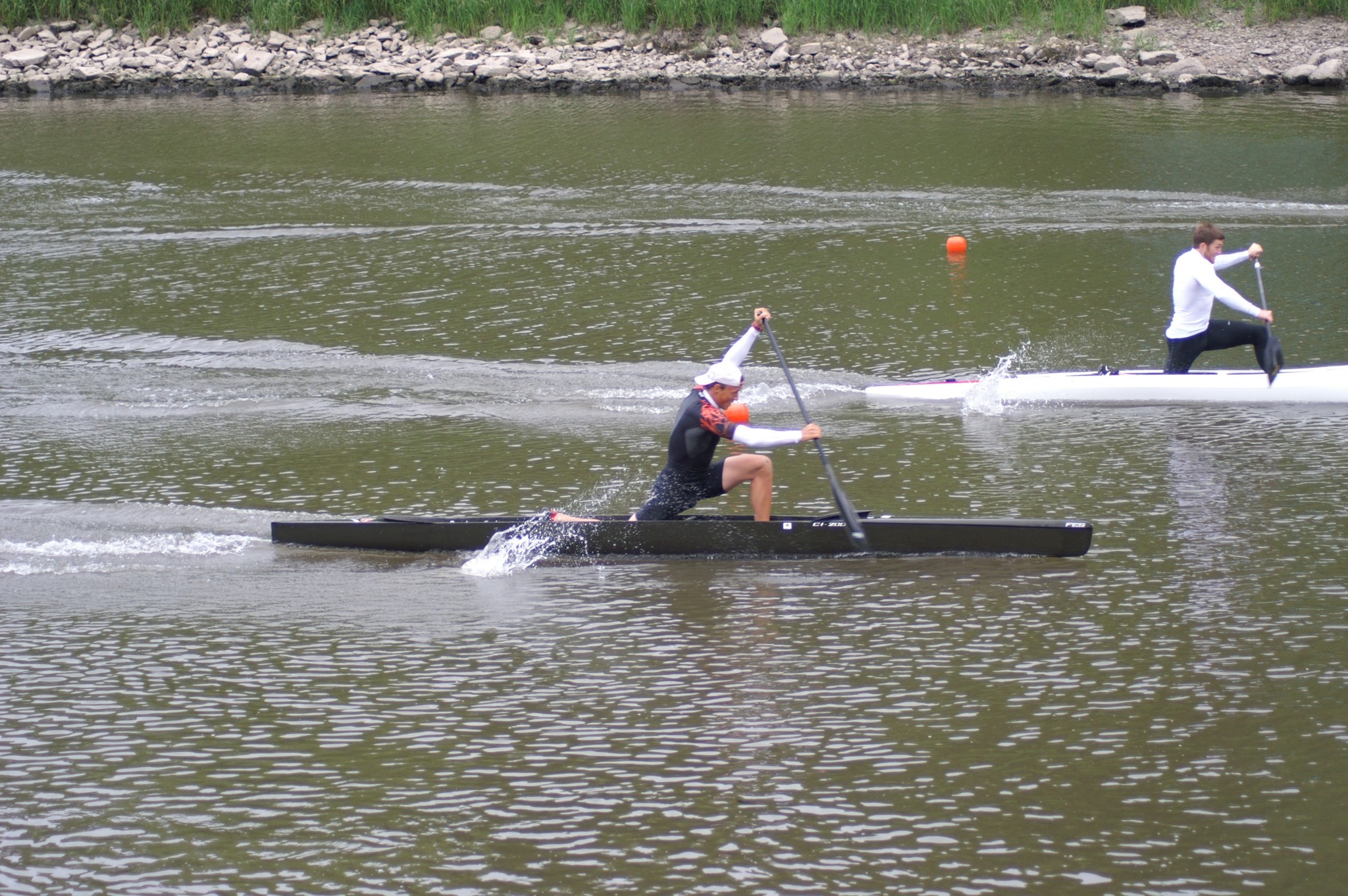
[751, 321, 763, 332]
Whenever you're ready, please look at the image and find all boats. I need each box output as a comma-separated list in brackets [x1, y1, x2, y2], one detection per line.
[864, 365, 1348, 407]
[271, 511, 1094, 558]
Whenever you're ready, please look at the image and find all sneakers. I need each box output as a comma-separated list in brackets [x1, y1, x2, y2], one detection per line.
[1097, 364, 1119, 375]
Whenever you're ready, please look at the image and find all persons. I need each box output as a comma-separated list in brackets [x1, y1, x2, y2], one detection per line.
[550, 307, 822, 521]
[1097, 222, 1285, 376]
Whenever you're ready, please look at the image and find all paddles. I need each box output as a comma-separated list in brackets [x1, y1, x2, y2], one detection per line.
[758, 312, 871, 552]
[1252, 252, 1285, 388]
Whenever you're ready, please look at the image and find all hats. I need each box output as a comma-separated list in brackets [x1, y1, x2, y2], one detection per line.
[694, 363, 745, 386]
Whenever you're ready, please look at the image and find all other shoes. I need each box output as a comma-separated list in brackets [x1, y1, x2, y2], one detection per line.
[538, 508, 558, 522]
